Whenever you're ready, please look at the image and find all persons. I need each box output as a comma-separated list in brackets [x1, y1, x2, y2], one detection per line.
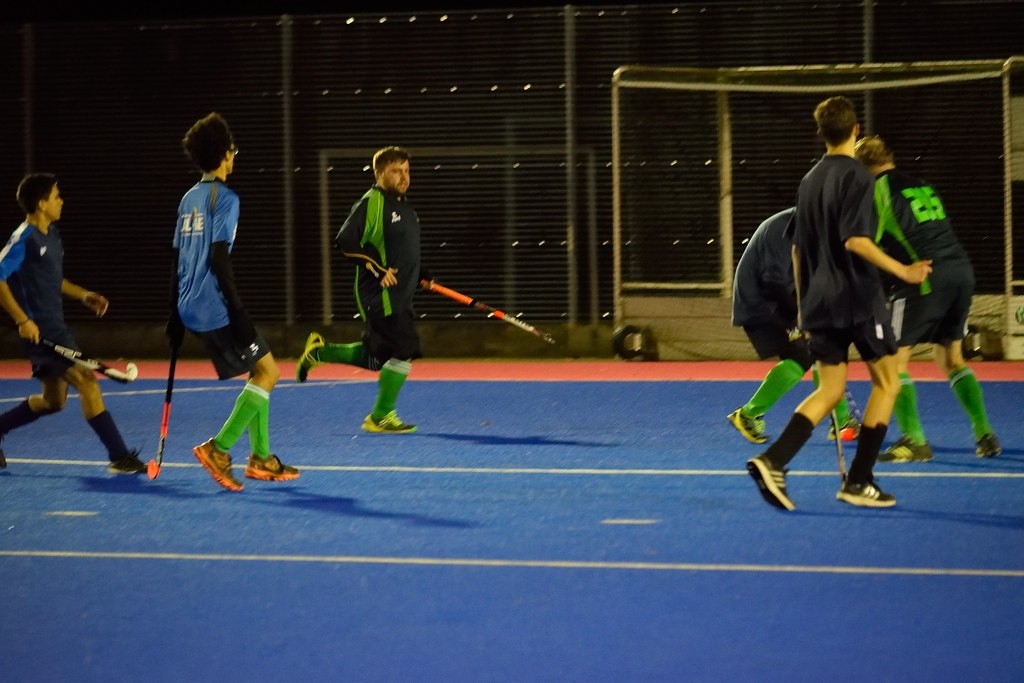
[163, 112, 299, 490]
[747, 96, 933, 513]
[297, 147, 435, 434]
[0, 173, 149, 474]
[854, 137, 1002, 462]
[729, 205, 861, 445]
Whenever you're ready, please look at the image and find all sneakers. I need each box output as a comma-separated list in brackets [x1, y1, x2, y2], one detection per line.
[246, 453, 299, 481]
[748, 455, 798, 511]
[836, 480, 896, 507]
[975, 432, 1002, 458]
[194, 438, 244, 491]
[727, 407, 769, 444]
[878, 436, 934, 462]
[296, 331, 326, 382]
[0, 434, 7, 467]
[361, 410, 415, 434]
[108, 448, 146, 473]
[828, 416, 862, 441]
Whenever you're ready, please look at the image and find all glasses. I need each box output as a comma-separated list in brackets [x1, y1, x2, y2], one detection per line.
[228, 145, 240, 156]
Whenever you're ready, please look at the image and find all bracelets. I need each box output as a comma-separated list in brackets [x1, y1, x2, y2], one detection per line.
[16, 316, 29, 325]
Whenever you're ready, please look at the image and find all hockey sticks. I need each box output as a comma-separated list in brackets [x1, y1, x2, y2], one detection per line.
[419, 277, 557, 344]
[147, 346, 179, 480]
[845, 385, 862, 420]
[789, 243, 848, 485]
[38, 335, 139, 385]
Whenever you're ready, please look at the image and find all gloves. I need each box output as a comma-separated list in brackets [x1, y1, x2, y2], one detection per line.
[228, 308, 257, 350]
[166, 306, 186, 348]
